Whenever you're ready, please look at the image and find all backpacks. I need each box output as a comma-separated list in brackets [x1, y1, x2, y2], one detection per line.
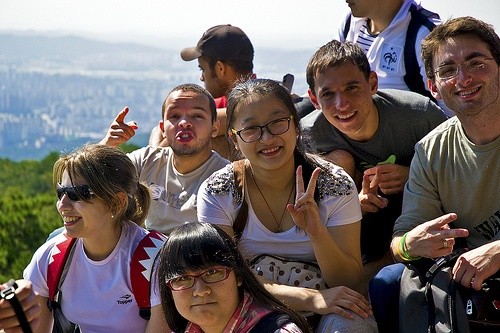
[398, 249, 499, 333]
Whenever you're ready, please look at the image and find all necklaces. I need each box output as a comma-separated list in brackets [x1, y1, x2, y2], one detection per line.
[250, 163, 297, 233]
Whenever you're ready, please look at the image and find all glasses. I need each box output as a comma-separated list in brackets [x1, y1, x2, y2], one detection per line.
[165, 266, 234, 291]
[231, 115, 294, 143]
[433, 56, 496, 79]
[56, 182, 99, 202]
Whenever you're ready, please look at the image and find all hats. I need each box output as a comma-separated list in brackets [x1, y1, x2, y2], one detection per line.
[180, 24, 254, 69]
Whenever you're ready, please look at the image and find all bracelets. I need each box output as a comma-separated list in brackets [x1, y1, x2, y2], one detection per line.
[397, 232, 422, 262]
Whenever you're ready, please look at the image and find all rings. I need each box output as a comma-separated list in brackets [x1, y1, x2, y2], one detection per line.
[442, 240, 448, 249]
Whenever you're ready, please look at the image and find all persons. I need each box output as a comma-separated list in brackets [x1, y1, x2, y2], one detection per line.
[97, 84, 233, 237]
[294, 40, 451, 266]
[368, 17, 500, 333]
[0, 144, 173, 333]
[149, 25, 256, 161]
[294, 0, 456, 122]
[197, 79, 379, 333]
[159, 221, 313, 333]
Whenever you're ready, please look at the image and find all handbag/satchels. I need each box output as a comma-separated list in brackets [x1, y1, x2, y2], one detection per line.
[249, 252, 325, 330]
